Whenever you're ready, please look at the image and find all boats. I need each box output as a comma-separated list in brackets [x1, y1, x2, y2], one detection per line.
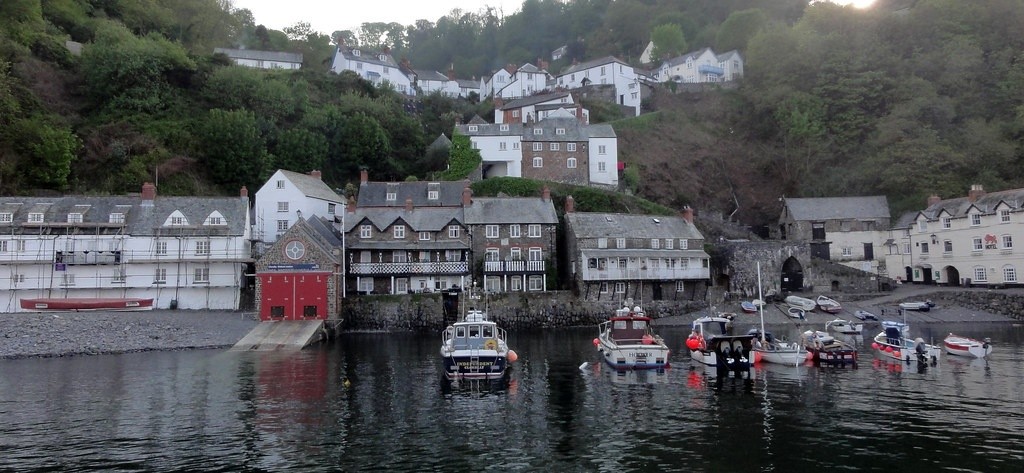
[942, 332, 994, 357]
[740, 301, 761, 313]
[592, 256, 674, 374]
[899, 302, 932, 312]
[438, 279, 519, 382]
[880, 321, 910, 333]
[825, 318, 869, 334]
[787, 307, 806, 320]
[874, 306, 946, 365]
[800, 330, 860, 366]
[685, 290, 762, 380]
[783, 295, 821, 312]
[752, 299, 767, 309]
[816, 295, 845, 314]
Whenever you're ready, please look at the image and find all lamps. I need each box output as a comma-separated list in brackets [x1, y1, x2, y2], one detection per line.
[930, 233, 938, 244]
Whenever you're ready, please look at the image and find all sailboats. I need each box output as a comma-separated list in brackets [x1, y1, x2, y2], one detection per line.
[744, 261, 814, 367]
[14, 235, 156, 311]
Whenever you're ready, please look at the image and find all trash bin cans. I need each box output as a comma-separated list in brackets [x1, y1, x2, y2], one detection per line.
[170, 300, 176, 309]
